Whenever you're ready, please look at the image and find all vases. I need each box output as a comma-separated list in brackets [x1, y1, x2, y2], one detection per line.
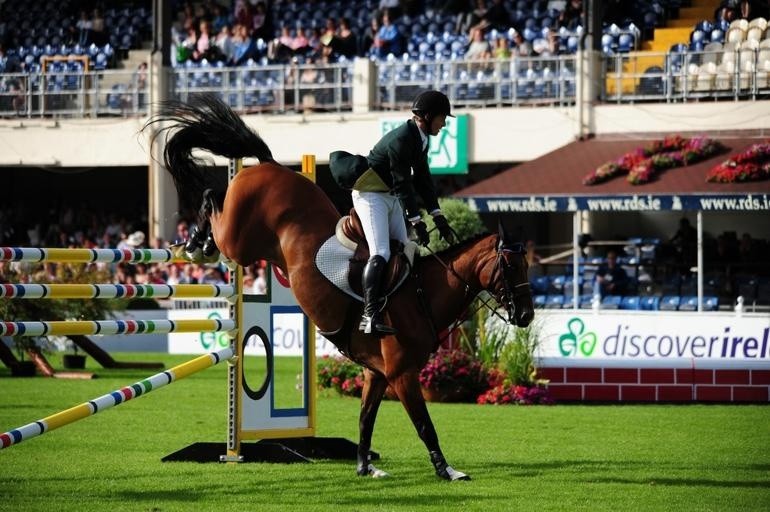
[421, 384, 462, 400]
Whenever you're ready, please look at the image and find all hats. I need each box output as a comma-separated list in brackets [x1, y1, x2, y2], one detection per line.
[410, 92, 456, 119]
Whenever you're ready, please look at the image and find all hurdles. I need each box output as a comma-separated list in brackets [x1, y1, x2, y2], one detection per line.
[0, 242, 244, 462]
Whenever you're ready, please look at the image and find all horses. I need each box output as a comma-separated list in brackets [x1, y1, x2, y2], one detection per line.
[135, 91, 535, 482]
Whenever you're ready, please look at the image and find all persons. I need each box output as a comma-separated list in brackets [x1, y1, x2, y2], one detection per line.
[716, 2, 770, 23]
[328, 91, 454, 336]
[0, 1, 145, 119]
[523, 217, 770, 313]
[0, 193, 226, 250]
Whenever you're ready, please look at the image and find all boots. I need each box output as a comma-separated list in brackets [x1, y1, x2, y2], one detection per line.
[359, 286, 399, 335]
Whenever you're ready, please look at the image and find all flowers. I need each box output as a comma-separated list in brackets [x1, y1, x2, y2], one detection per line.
[419, 345, 481, 401]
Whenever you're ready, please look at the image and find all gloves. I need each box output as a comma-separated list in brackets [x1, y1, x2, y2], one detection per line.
[413, 221, 430, 248]
[432, 215, 452, 241]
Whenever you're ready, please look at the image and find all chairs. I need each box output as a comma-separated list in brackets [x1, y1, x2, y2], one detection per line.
[0, 1, 149, 99]
[168, 1, 636, 115]
[532, 237, 719, 312]
[639, 0, 770, 95]
